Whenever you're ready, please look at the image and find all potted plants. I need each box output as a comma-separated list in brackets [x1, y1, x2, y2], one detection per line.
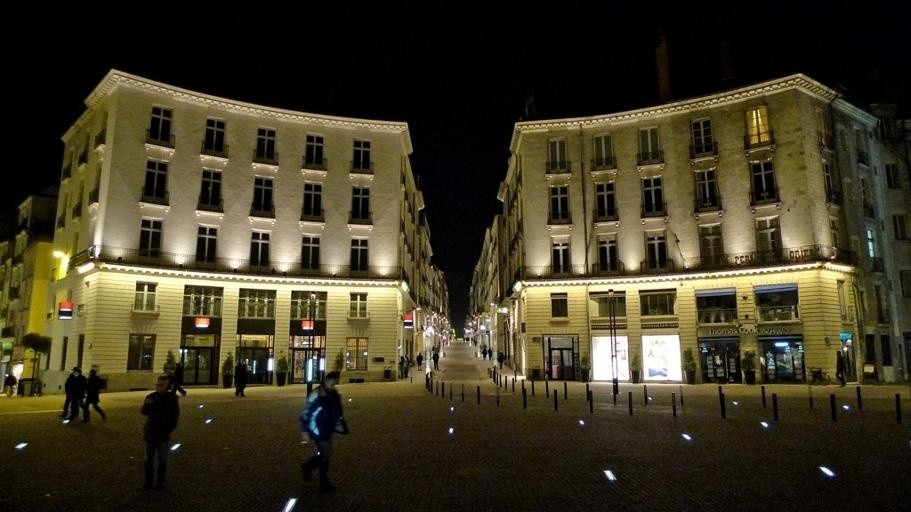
[222, 350, 234, 388]
[163, 350, 178, 390]
[741, 350, 756, 385]
[682, 348, 697, 385]
[580, 352, 591, 382]
[331, 347, 345, 384]
[630, 355, 641, 384]
[276, 354, 289, 386]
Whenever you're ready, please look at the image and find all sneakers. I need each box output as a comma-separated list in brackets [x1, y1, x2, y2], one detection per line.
[299, 463, 314, 481]
[319, 480, 336, 494]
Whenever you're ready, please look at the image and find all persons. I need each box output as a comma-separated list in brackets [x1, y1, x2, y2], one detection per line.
[417, 353, 424, 370]
[405, 355, 410, 377]
[170, 362, 187, 397]
[59, 366, 107, 424]
[299, 371, 349, 491]
[488, 349, 493, 360]
[399, 356, 406, 378]
[140, 375, 180, 488]
[837, 350, 846, 386]
[235, 359, 247, 396]
[433, 351, 439, 369]
[5, 372, 17, 398]
[482, 348, 488, 359]
[498, 352, 505, 368]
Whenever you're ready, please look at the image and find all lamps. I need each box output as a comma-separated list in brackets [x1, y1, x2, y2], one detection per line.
[537, 256, 741, 280]
[117, 257, 336, 278]
